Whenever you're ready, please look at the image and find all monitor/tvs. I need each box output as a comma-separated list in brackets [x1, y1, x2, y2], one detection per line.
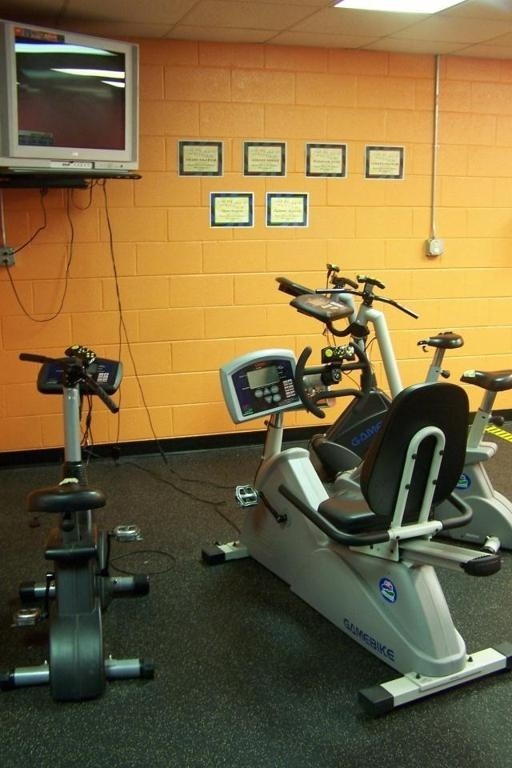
[0, 20, 140, 172]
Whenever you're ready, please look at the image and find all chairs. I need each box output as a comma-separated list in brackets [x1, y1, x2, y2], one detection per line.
[279, 382, 473, 546]
